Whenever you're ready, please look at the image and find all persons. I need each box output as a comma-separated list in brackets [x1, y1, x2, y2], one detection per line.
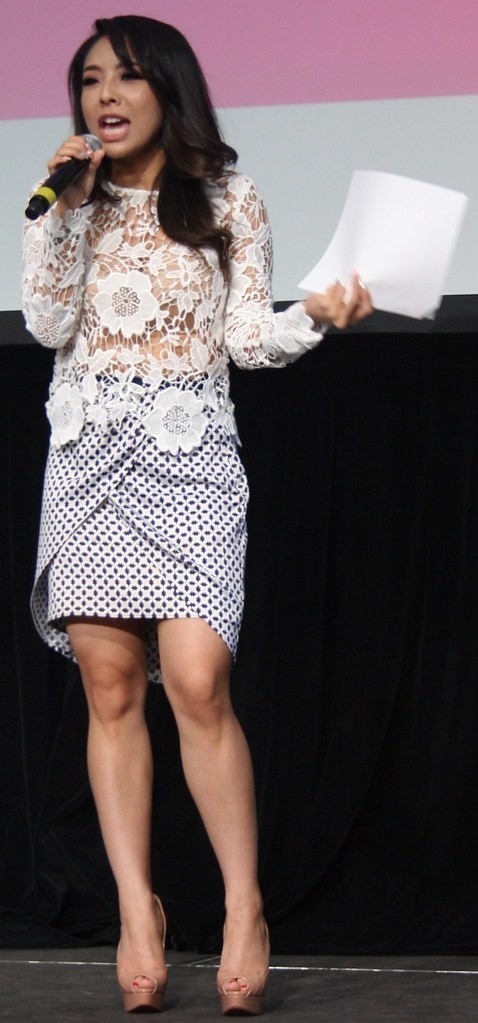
[21, 17, 374, 1014]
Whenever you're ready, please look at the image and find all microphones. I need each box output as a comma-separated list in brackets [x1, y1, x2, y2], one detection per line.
[26, 135, 102, 221]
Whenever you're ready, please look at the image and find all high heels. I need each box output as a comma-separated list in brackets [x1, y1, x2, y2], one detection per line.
[116, 893, 167, 1013]
[216, 914, 270, 1015]
